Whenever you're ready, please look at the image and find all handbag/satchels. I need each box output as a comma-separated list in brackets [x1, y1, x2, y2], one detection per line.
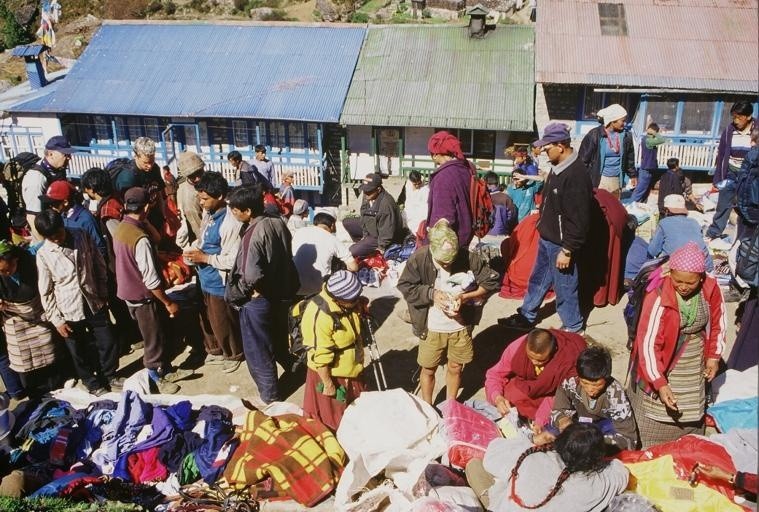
[465, 160, 497, 239]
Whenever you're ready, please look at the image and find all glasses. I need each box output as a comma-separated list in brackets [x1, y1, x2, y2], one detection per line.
[334, 271, 363, 304]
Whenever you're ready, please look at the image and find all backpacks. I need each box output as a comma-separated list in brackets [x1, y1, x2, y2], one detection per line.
[0, 152, 53, 228]
[624, 255, 669, 394]
[105, 157, 142, 193]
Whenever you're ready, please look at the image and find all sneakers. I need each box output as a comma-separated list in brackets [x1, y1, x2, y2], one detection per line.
[396, 309, 413, 324]
[221, 360, 241, 373]
[367, 267, 380, 288]
[358, 267, 370, 286]
[148, 377, 181, 394]
[104, 377, 127, 392]
[161, 366, 194, 383]
[497, 307, 535, 331]
[204, 353, 226, 365]
[89, 385, 112, 397]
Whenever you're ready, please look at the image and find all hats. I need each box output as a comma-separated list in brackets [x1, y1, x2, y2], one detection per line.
[37, 180, 76, 208]
[44, 136, 81, 155]
[512, 144, 529, 157]
[177, 152, 205, 179]
[124, 186, 151, 214]
[313, 207, 337, 233]
[533, 121, 572, 147]
[292, 199, 309, 215]
[326, 269, 362, 300]
[669, 241, 706, 273]
[357, 174, 382, 193]
[664, 193, 689, 215]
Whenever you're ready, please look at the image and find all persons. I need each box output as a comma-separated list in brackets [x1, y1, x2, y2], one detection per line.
[1, 98, 758, 512]
